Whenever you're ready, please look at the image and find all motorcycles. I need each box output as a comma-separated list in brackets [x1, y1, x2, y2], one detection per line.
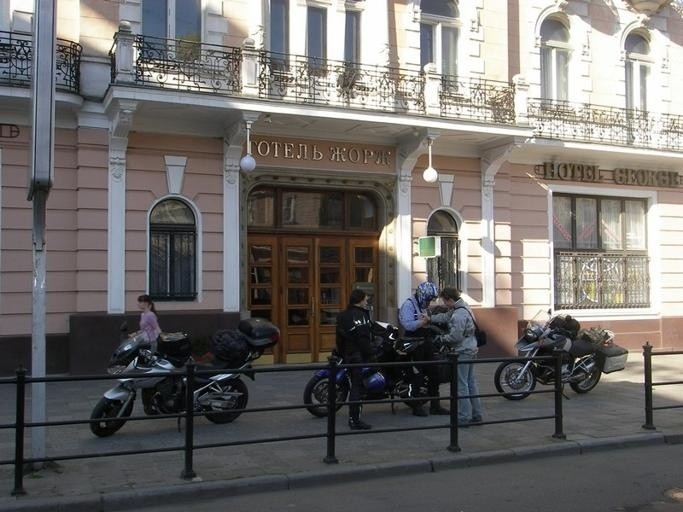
[493, 308, 615, 401]
[89, 318, 280, 438]
[302, 319, 447, 418]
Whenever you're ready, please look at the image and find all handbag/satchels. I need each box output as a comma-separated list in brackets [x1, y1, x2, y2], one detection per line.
[475, 329, 486, 347]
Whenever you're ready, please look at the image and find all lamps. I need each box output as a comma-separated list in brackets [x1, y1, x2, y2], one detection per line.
[239, 121, 256, 173]
[422, 136, 438, 183]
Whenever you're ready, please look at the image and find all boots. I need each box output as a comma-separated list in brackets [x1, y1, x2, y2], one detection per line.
[348, 410, 371, 429]
[412, 400, 428, 417]
[429, 400, 450, 415]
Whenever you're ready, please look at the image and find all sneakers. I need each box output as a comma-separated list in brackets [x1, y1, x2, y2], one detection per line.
[472, 415, 481, 422]
[458, 416, 469, 425]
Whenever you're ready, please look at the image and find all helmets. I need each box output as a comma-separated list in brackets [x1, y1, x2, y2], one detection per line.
[360, 367, 385, 393]
[416, 282, 438, 310]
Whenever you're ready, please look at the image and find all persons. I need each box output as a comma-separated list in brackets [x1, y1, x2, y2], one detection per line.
[126, 295, 163, 357]
[335, 291, 373, 431]
[398, 281, 451, 417]
[418, 287, 484, 425]
